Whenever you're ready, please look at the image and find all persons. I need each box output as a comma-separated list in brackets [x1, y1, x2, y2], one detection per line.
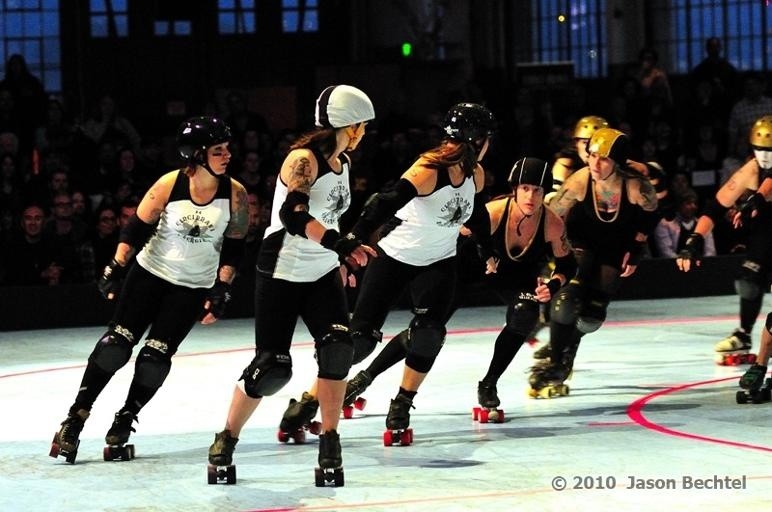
[1, 36, 771, 287]
[205, 82, 377, 469]
[345, 157, 578, 412]
[280, 101, 493, 434]
[523, 127, 677, 390]
[58, 115, 251, 452]
[522, 112, 612, 340]
[673, 112, 772, 354]
[732, 167, 772, 389]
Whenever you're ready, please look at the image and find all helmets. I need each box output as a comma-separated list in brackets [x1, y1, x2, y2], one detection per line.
[507, 157, 553, 193]
[442, 103, 496, 142]
[315, 85, 375, 127]
[586, 127, 628, 163]
[573, 116, 609, 139]
[176, 116, 231, 159]
[750, 115, 772, 151]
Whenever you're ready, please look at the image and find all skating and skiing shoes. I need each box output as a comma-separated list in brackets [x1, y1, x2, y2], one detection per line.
[49, 328, 772, 487]
